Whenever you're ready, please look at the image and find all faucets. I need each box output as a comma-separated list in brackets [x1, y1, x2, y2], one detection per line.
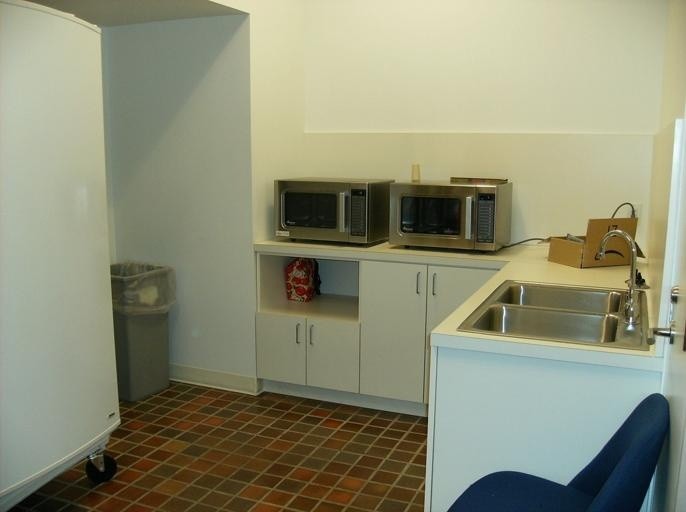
[596, 229, 638, 299]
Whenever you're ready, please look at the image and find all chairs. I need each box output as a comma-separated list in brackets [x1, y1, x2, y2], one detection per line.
[447, 392, 671, 511]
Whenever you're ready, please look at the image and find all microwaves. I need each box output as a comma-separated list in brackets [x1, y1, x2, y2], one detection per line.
[273, 176, 394, 248]
[385, 180, 512, 252]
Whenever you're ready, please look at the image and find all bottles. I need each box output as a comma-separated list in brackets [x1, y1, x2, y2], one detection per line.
[411, 163, 421, 181]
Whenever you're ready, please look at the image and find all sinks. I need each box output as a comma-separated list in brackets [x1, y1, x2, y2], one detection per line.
[497, 284, 608, 314]
[474, 305, 605, 344]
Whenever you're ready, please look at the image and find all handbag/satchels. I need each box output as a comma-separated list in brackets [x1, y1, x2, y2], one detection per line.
[285, 257, 321, 303]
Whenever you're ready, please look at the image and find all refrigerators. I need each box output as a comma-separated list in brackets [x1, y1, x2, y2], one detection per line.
[1, 0, 121, 507]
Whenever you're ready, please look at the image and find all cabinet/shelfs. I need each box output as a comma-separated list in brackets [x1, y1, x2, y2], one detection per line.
[360, 263, 499, 402]
[256, 295, 360, 397]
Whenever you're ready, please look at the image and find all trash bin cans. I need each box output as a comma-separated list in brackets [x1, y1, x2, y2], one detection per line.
[110, 262, 170, 402]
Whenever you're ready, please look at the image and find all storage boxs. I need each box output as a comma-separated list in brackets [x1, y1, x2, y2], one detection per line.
[539, 218, 640, 269]
